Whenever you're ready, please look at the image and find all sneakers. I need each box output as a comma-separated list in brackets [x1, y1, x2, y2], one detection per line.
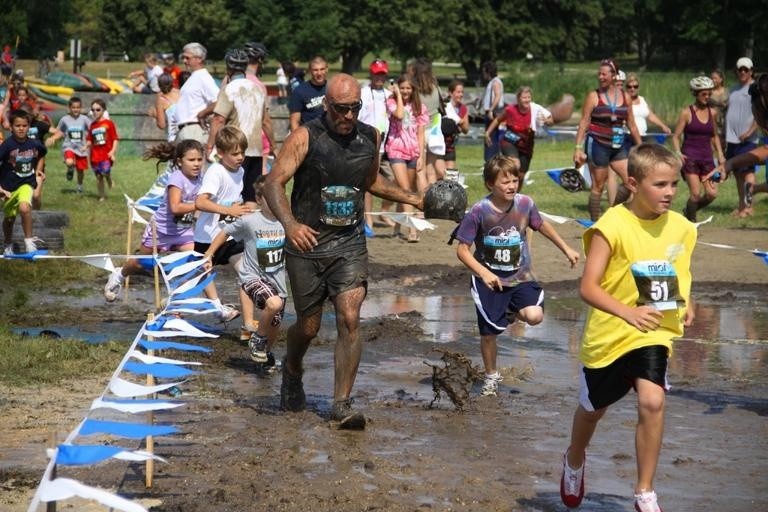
[281, 356, 309, 414]
[634, 492, 662, 512]
[248, 335, 270, 365]
[4, 244, 13, 259]
[27, 239, 41, 256]
[408, 233, 420, 243]
[106, 268, 121, 303]
[481, 373, 503, 398]
[391, 228, 401, 242]
[561, 452, 586, 507]
[215, 304, 239, 324]
[330, 401, 367, 428]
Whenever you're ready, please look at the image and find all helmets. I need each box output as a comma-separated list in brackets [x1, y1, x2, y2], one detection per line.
[688, 76, 715, 95]
[424, 180, 468, 223]
[225, 49, 249, 71]
[560, 163, 583, 192]
[244, 42, 268, 60]
[616, 70, 627, 80]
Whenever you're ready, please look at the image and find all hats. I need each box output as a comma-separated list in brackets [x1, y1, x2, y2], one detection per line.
[371, 60, 389, 74]
[737, 57, 753, 70]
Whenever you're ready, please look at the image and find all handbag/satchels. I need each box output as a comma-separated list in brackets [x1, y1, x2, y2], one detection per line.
[489, 87, 506, 119]
[437, 84, 458, 135]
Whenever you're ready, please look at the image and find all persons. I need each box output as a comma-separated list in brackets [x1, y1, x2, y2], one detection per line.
[286, 57, 330, 131]
[197, 126, 262, 343]
[85, 99, 119, 205]
[610, 68, 627, 90]
[560, 143, 698, 512]
[384, 74, 426, 243]
[459, 155, 579, 396]
[701, 71, 768, 184]
[672, 76, 725, 225]
[103, 140, 241, 325]
[204, 48, 267, 204]
[429, 107, 459, 181]
[127, 55, 163, 92]
[437, 81, 467, 169]
[221, 43, 281, 200]
[2, 65, 53, 118]
[178, 42, 219, 142]
[261, 73, 428, 430]
[204, 174, 292, 374]
[574, 60, 640, 223]
[1, 111, 46, 258]
[482, 63, 504, 160]
[357, 61, 402, 238]
[159, 54, 181, 88]
[707, 68, 728, 179]
[148, 72, 179, 142]
[723, 57, 768, 217]
[484, 86, 552, 193]
[276, 58, 306, 93]
[397, 58, 448, 215]
[606, 74, 672, 207]
[58, 98, 89, 185]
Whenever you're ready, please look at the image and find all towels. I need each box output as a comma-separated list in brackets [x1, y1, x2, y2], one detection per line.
[530, 101, 551, 131]
[444, 102, 461, 125]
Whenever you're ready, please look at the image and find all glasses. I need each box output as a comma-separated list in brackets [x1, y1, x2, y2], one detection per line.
[92, 108, 102, 112]
[13, 81, 22, 85]
[182, 55, 192, 59]
[625, 84, 640, 88]
[329, 99, 365, 113]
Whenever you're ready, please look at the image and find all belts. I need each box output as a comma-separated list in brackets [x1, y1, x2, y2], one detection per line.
[176, 121, 201, 128]
